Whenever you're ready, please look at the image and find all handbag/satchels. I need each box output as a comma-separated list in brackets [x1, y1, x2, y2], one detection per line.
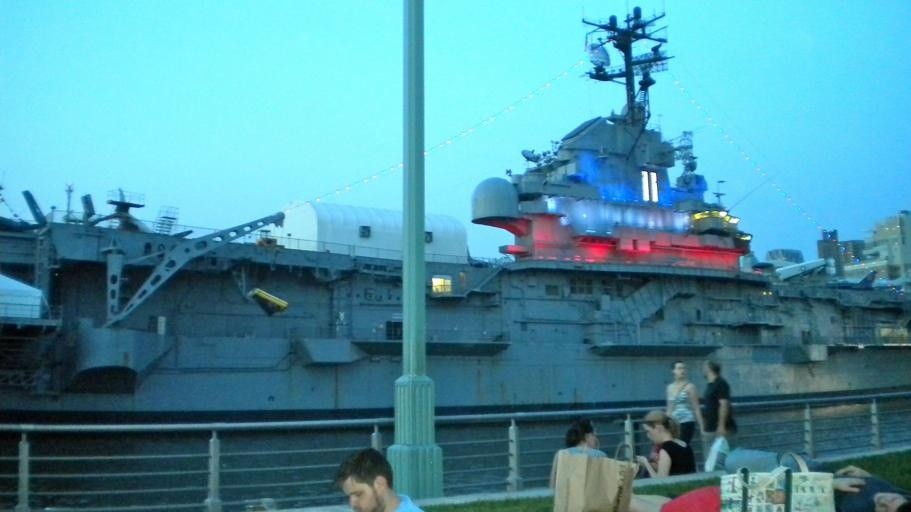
[720, 453, 836, 512]
[670, 418, 682, 438]
[553, 438, 638, 511]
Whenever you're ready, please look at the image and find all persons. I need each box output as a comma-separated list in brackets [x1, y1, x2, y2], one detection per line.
[666, 360, 703, 445]
[702, 360, 737, 470]
[332, 449, 424, 512]
[563, 416, 607, 458]
[633, 410, 699, 480]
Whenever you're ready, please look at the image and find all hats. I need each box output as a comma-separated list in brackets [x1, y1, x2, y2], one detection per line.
[644, 409, 667, 422]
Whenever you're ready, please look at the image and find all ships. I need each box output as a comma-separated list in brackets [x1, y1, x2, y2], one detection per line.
[0, 8, 911, 512]
